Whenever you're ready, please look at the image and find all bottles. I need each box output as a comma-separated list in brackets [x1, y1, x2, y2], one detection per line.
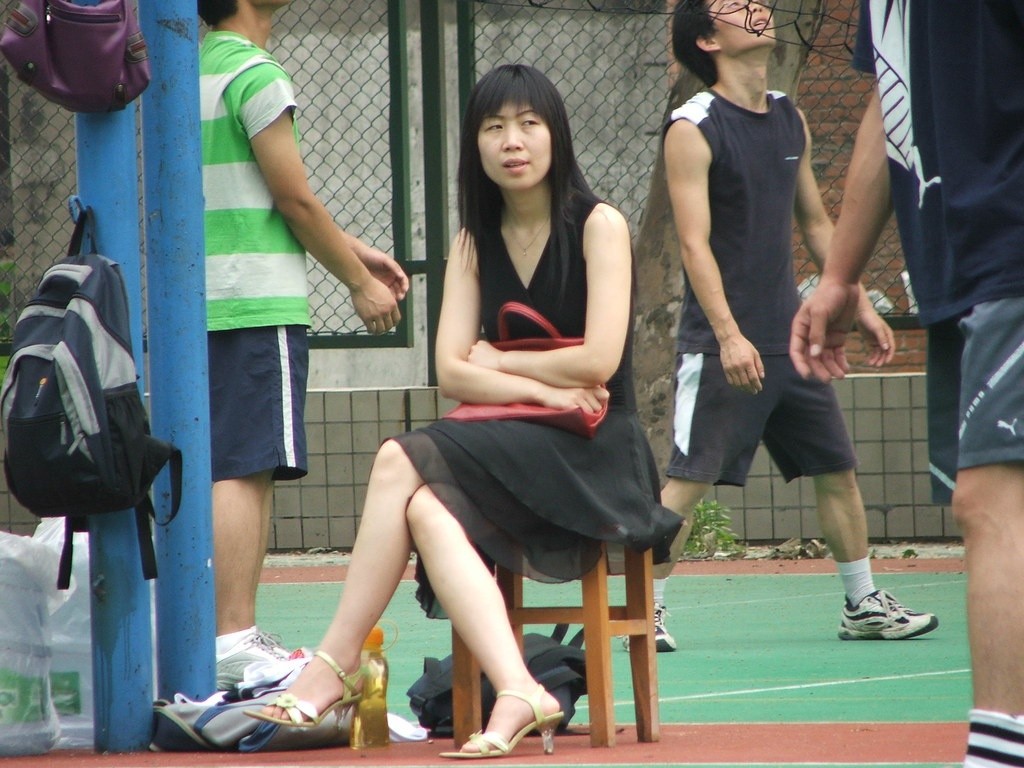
[0, 531, 95, 756]
[349, 619, 398, 751]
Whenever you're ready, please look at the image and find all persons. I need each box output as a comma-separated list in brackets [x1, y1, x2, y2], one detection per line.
[790, 0, 1024, 768]
[246, 66, 686, 759]
[618, 0, 940, 652]
[198, 0, 411, 691]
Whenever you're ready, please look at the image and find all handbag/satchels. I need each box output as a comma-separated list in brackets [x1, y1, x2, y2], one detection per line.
[4, 2, 150, 115]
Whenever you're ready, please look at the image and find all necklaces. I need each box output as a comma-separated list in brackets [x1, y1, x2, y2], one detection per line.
[510, 214, 549, 255]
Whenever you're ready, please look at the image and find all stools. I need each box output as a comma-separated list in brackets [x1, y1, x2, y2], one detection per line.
[451, 541, 659, 750]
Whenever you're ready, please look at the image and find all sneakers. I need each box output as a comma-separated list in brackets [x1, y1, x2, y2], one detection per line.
[216, 626, 296, 689]
[840, 588, 939, 638]
[623, 604, 680, 652]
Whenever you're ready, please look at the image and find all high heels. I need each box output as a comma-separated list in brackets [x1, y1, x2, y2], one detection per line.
[440, 686, 574, 754]
[252, 651, 369, 732]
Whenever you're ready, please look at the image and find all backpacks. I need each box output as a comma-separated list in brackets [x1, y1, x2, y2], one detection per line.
[407, 621, 591, 734]
[0, 206, 184, 592]
